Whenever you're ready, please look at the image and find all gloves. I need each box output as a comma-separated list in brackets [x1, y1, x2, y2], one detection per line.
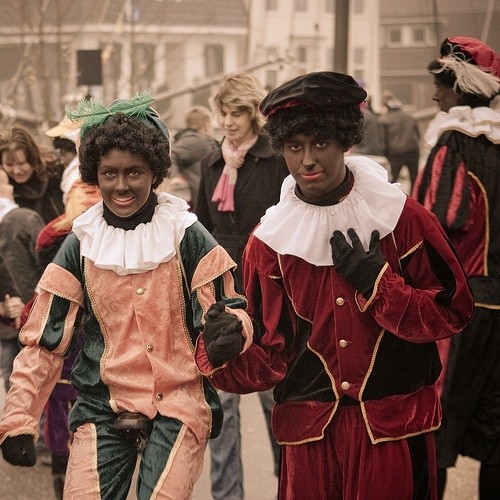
[329, 228, 386, 300]
[202, 303, 243, 364]
[1, 434, 36, 466]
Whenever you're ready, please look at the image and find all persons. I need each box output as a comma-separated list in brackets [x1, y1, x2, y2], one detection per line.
[194, 72, 475, 500]
[0, 35, 500, 500]
[1, 88, 253, 500]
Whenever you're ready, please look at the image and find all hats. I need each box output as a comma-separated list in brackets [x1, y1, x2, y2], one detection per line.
[258, 72, 368, 117]
[44, 108, 90, 144]
[439, 36, 500, 78]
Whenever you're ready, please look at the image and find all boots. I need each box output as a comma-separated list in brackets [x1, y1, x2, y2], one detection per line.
[51, 454, 69, 500]
